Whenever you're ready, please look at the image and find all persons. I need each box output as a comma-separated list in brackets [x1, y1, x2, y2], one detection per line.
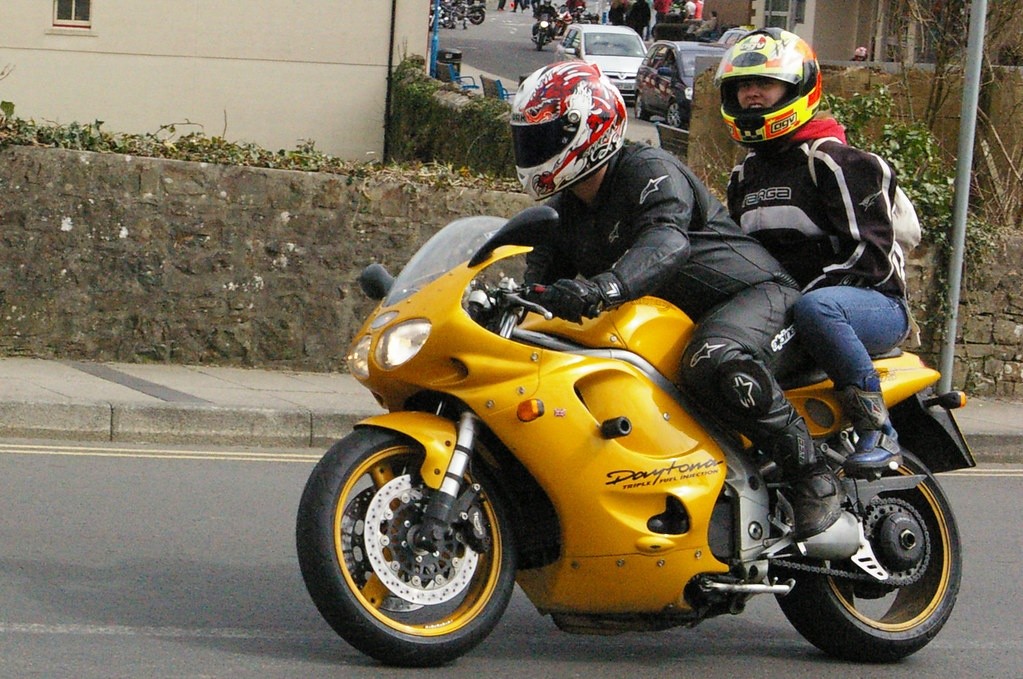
[848, 46, 870, 62]
[464, 0, 718, 41]
[711, 26, 920, 477]
[508, 58, 849, 537]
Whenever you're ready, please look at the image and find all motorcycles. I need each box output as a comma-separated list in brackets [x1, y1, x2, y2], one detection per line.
[292, 203, 983, 664]
[427, 0, 597, 54]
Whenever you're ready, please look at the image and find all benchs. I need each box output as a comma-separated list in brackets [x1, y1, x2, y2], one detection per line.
[480, 73, 516, 103]
[654, 121, 689, 161]
[435, 60, 479, 91]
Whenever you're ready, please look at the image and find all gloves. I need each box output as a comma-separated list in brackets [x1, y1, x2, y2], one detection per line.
[538, 278, 605, 326]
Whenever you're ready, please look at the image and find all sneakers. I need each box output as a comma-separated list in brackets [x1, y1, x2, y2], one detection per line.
[790, 465, 843, 539]
[842, 426, 904, 475]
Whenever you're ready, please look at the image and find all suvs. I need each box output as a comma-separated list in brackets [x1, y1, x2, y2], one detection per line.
[718, 27, 758, 52]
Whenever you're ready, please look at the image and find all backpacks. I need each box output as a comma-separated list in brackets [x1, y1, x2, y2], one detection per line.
[890, 185, 924, 262]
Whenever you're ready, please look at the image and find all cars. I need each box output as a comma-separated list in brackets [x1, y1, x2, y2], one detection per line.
[631, 35, 731, 132]
[552, 21, 653, 109]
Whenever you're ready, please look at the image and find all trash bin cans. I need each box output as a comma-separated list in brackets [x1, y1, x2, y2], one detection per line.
[437, 48, 462, 82]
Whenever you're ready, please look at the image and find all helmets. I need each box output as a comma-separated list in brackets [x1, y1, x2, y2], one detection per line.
[509, 61, 628, 202]
[715, 26, 824, 148]
